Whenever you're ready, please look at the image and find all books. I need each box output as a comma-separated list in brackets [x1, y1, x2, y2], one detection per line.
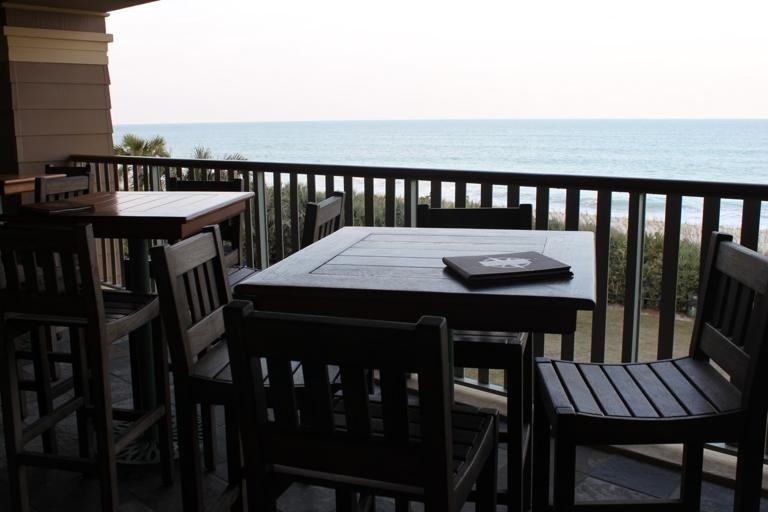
[18, 199, 95, 216]
[441, 250, 575, 285]
